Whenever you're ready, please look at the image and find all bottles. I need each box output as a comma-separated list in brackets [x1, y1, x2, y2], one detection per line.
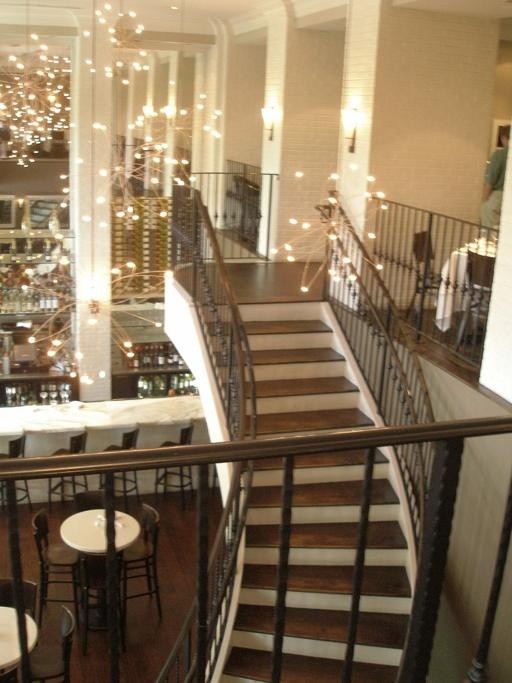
[9, 238, 52, 262]
[1, 353, 10, 377]
[3, 384, 72, 406]
[124, 342, 196, 399]
[0, 286, 58, 312]
[69, 362, 77, 378]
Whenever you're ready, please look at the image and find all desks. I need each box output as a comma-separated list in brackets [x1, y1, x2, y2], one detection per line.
[2, 607, 37, 675]
[65, 507, 139, 556]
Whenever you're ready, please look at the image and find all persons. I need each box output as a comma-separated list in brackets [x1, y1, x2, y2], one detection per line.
[473, 123, 510, 240]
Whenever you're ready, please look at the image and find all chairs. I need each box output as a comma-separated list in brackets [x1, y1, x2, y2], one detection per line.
[34, 606, 76, 683]
[73, 486, 113, 508]
[4, 578, 39, 611]
[70, 561, 127, 658]
[27, 508, 73, 629]
[122, 504, 167, 618]
[407, 229, 495, 354]
